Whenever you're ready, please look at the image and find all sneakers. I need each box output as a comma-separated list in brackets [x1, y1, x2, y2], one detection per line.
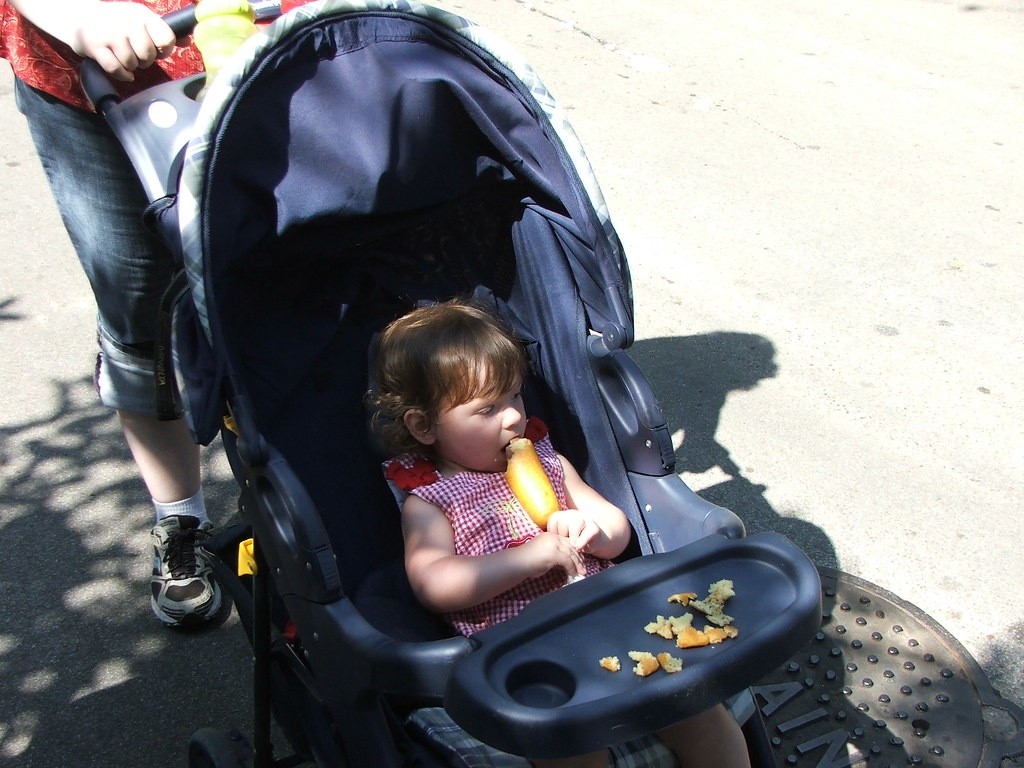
[150, 514, 222, 625]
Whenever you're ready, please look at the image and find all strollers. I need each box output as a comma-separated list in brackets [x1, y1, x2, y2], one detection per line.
[57, 1, 823, 768]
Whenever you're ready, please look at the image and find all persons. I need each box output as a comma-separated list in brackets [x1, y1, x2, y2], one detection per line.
[369, 304, 750, 768]
[0, 0, 325, 635]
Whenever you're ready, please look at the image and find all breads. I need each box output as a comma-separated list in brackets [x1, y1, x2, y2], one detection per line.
[505, 438, 560, 529]
